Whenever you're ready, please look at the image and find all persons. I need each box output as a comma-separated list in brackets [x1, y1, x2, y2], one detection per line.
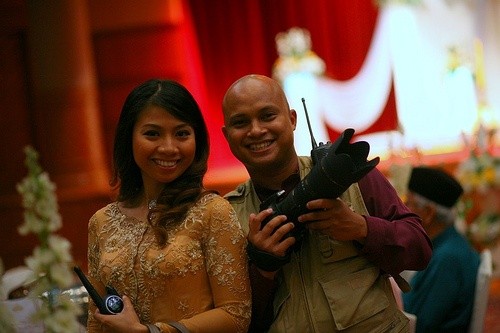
[401, 165, 480, 333]
[87, 78, 252, 333]
[222, 75, 433, 333]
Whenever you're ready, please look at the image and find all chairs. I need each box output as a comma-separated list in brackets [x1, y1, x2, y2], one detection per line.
[470, 249, 492, 333]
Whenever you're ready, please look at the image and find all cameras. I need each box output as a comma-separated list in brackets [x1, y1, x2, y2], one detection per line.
[259, 129, 380, 250]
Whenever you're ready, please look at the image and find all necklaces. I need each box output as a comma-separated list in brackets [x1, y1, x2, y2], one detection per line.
[146, 198, 159, 219]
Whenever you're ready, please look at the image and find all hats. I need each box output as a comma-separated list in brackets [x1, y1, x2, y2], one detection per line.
[407, 166, 464, 209]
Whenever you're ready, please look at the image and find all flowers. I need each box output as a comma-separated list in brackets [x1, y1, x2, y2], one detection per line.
[17, 146, 85, 333]
